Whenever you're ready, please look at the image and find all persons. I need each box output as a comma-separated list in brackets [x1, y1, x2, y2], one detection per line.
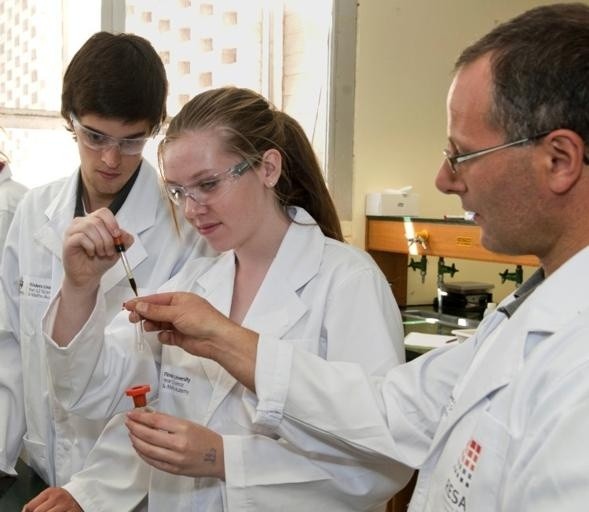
[125, 4, 589, 512]
[42, 87, 415, 512]
[0, 31, 223, 512]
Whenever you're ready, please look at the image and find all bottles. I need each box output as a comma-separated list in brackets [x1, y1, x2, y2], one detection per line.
[133, 299, 145, 354]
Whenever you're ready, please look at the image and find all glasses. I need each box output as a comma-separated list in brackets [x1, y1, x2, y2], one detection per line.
[439, 128, 589, 175]
[164, 151, 262, 209]
[69, 108, 163, 155]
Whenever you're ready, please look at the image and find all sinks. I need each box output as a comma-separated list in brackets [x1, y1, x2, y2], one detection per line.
[404, 310, 481, 328]
